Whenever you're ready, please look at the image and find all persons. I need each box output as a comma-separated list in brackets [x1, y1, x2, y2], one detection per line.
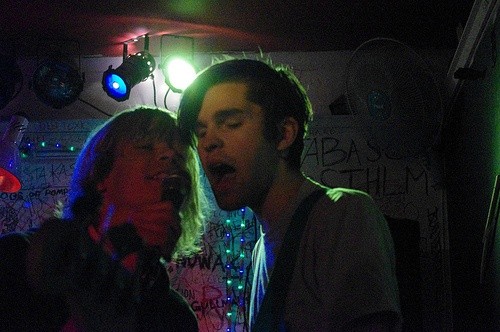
[1, 108, 201, 332]
[171, 56, 404, 332]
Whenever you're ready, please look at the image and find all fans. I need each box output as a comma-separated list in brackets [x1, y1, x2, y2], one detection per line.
[329, 36, 450, 164]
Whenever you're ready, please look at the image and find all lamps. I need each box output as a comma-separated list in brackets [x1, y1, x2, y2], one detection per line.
[159, 30, 202, 94]
[27, 37, 86, 109]
[101, 32, 156, 103]
[0, 47, 24, 116]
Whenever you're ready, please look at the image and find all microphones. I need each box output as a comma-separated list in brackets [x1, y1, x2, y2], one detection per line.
[135, 170, 191, 284]
